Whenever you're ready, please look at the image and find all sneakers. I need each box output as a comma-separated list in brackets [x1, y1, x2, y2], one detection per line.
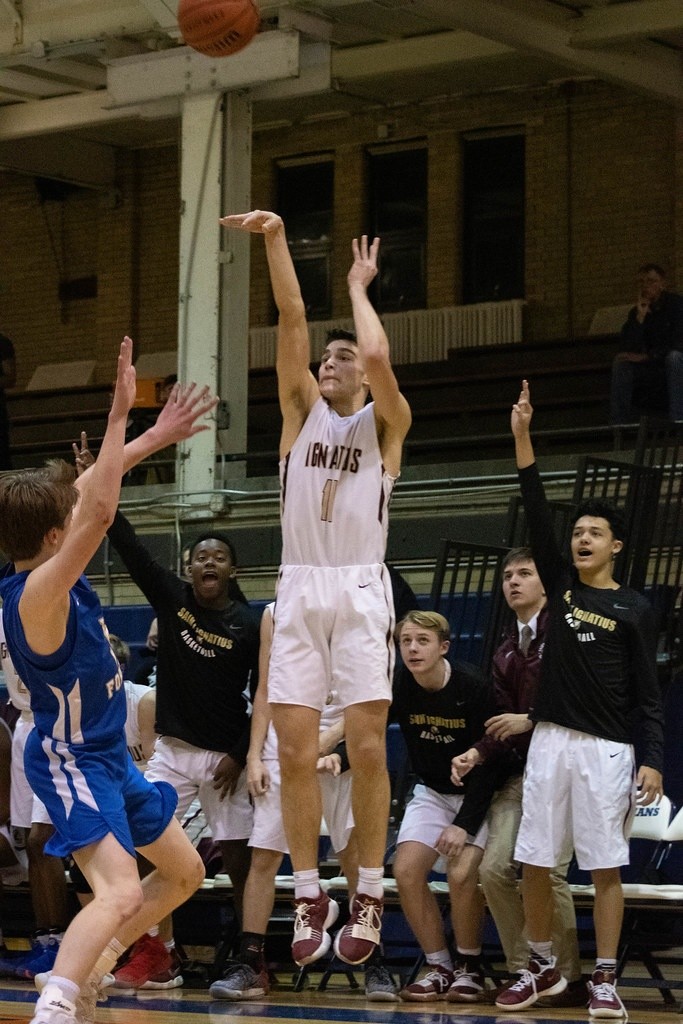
[291, 883, 340, 966]
[401, 964, 455, 1001]
[143, 960, 183, 990]
[496, 955, 568, 1010]
[445, 962, 487, 1002]
[587, 969, 629, 1019]
[0, 939, 45, 973]
[209, 963, 271, 999]
[335, 892, 383, 964]
[364, 965, 399, 1001]
[114, 932, 174, 989]
[17, 938, 60, 979]
[29, 987, 77, 1024]
[35, 970, 114, 1024]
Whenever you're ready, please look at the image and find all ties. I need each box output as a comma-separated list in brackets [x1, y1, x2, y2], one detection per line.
[521, 626, 532, 658]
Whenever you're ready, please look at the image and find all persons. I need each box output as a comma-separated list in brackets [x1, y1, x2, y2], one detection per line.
[448, 545, 582, 1009]
[0, 333, 16, 470]
[0, 336, 223, 1024]
[395, 608, 505, 1003]
[0, 631, 209, 991]
[121, 371, 178, 487]
[495, 379, 665, 1018]
[146, 541, 194, 687]
[72, 430, 260, 999]
[209, 207, 420, 1003]
[596, 264, 683, 438]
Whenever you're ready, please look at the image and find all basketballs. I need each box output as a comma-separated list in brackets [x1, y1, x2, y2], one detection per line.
[178, 0, 260, 58]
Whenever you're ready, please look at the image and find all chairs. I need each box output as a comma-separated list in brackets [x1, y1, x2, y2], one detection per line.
[0, 722, 683, 1005]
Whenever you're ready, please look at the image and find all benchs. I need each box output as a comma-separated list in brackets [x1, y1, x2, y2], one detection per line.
[3, 333, 683, 486]
[1, 585, 682, 722]
